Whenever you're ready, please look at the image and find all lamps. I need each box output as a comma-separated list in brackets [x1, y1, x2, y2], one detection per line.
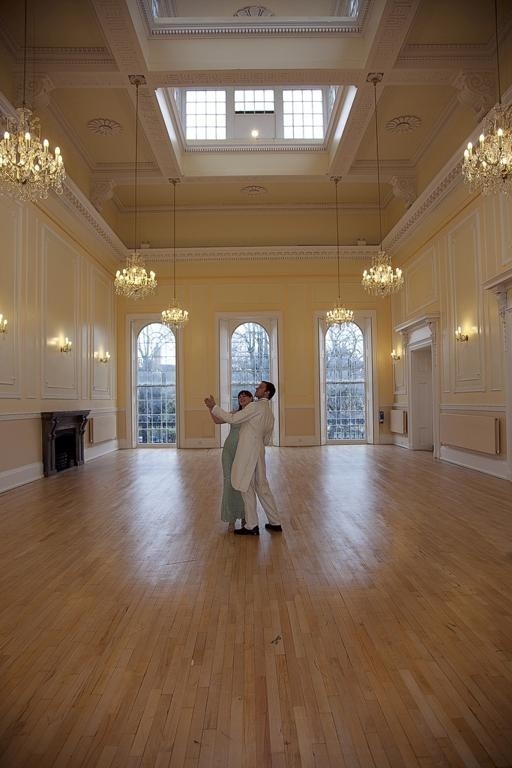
[113, 74, 191, 328]
[461, 0, 512, 201]
[324, 71, 407, 327]
[0, 0, 68, 204]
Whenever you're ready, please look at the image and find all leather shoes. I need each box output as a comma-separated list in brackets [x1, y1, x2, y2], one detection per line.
[232, 525, 260, 536]
[265, 524, 282, 532]
[227, 522, 235, 533]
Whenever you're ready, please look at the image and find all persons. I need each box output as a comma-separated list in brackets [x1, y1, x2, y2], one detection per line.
[201, 380, 283, 536]
[205, 390, 254, 534]
